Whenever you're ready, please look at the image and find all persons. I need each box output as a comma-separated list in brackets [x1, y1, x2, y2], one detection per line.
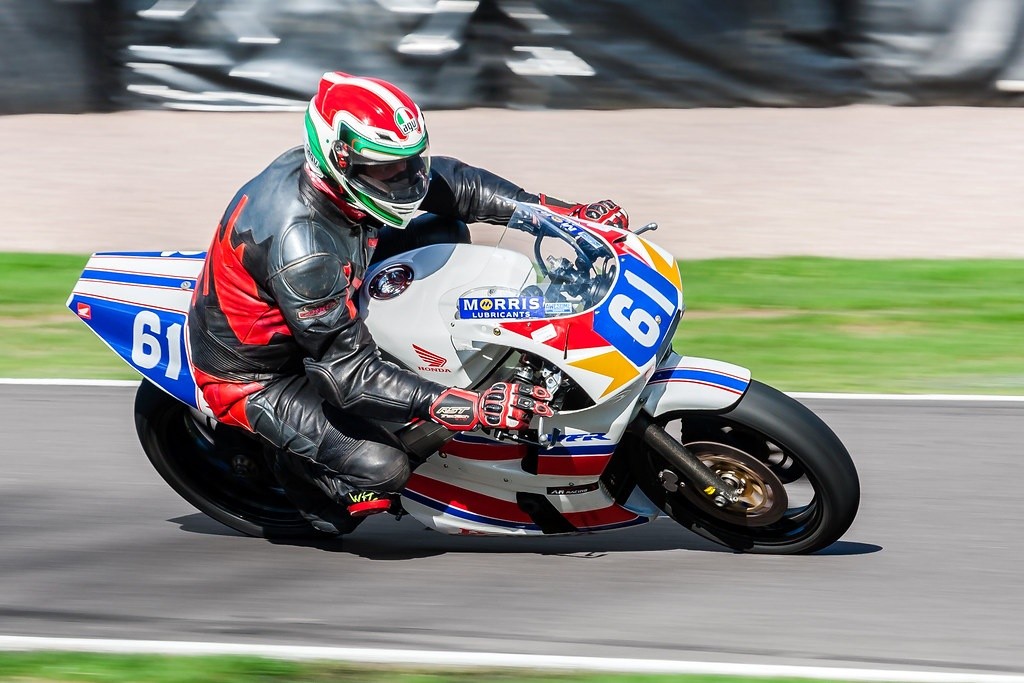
[176, 70, 631, 534]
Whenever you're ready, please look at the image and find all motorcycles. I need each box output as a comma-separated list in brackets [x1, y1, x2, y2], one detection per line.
[67, 202, 861, 555]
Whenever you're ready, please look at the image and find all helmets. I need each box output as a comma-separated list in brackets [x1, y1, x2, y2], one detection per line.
[303, 71, 432, 230]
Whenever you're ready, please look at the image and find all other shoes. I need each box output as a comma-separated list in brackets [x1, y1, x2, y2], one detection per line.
[276, 479, 357, 533]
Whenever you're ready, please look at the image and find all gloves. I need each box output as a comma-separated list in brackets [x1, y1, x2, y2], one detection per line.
[539, 191, 629, 231]
[429, 381, 553, 432]
